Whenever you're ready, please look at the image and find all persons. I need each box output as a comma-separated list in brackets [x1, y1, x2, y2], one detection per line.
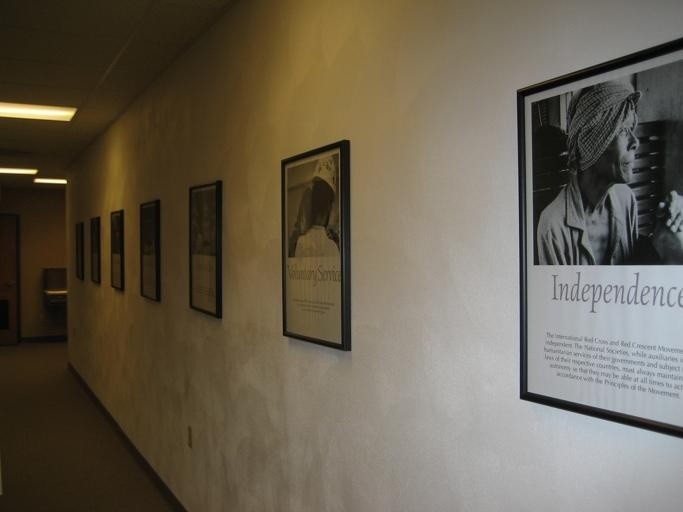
[293, 175, 340, 258]
[537, 80, 640, 266]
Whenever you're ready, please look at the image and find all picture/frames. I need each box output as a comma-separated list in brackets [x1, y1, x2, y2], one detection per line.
[281, 138, 350, 349]
[190, 181, 221, 317]
[74, 200, 159, 302]
[517, 37, 683, 436]
